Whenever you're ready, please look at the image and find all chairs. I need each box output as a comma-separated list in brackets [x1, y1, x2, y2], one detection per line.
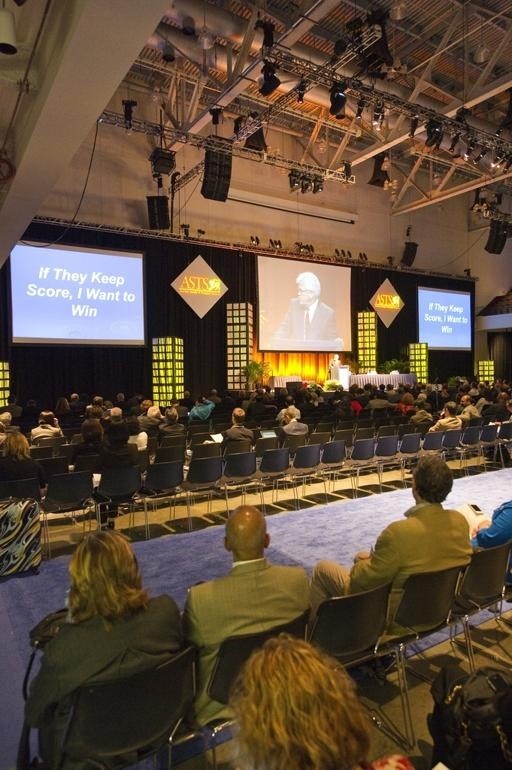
[282, 393, 510, 504]
[206, 607, 311, 770]
[0, 397, 289, 559]
[304, 580, 394, 671]
[455, 540, 511, 679]
[55, 645, 197, 769]
[370, 564, 476, 750]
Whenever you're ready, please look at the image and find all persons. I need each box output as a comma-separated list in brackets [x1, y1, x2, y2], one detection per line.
[330, 354, 344, 369]
[182, 505, 312, 732]
[274, 272, 337, 339]
[470, 497, 512, 604]
[426, 665, 512, 770]
[26, 530, 183, 769]
[310, 453, 473, 667]
[234, 634, 415, 770]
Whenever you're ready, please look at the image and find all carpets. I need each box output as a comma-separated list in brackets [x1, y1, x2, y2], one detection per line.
[0, 469, 511, 770]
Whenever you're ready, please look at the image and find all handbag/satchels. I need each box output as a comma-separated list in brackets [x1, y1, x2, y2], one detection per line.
[451, 666, 509, 733]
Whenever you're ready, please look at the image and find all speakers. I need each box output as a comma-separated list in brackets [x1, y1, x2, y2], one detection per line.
[367, 154, 389, 188]
[363, 20, 393, 79]
[234, 113, 267, 154]
[201, 135, 233, 202]
[152, 151, 174, 173]
[147, 195, 171, 230]
[484, 219, 508, 255]
[401, 242, 418, 265]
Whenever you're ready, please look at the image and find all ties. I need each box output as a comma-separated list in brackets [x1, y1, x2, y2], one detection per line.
[305, 307, 309, 335]
